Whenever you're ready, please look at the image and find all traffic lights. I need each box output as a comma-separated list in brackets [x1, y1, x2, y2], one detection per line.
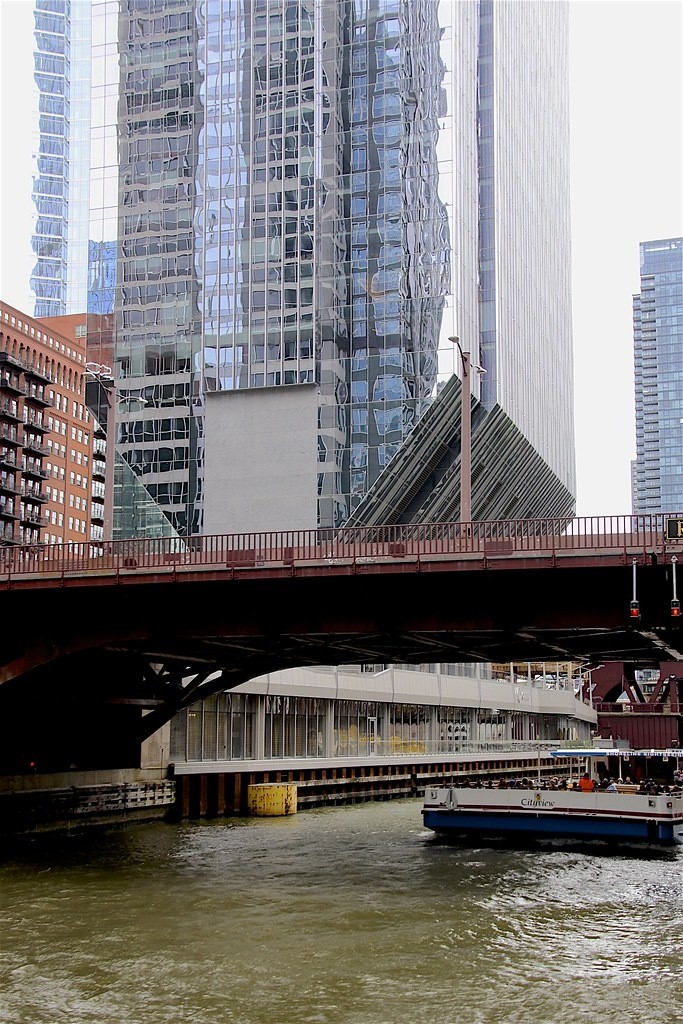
[631, 600, 640, 616]
[670, 599, 681, 616]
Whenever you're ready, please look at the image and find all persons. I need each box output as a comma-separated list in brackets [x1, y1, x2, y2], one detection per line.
[454, 777, 496, 789]
[579, 773, 594, 792]
[592, 770, 683, 797]
[570, 782, 581, 792]
[498, 776, 569, 791]
[439, 779, 450, 789]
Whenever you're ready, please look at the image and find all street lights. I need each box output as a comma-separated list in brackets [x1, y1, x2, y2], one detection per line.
[449, 335, 486, 538]
[82, 372, 147, 556]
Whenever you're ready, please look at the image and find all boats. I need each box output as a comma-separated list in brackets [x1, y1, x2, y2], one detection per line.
[420, 658, 683, 850]
